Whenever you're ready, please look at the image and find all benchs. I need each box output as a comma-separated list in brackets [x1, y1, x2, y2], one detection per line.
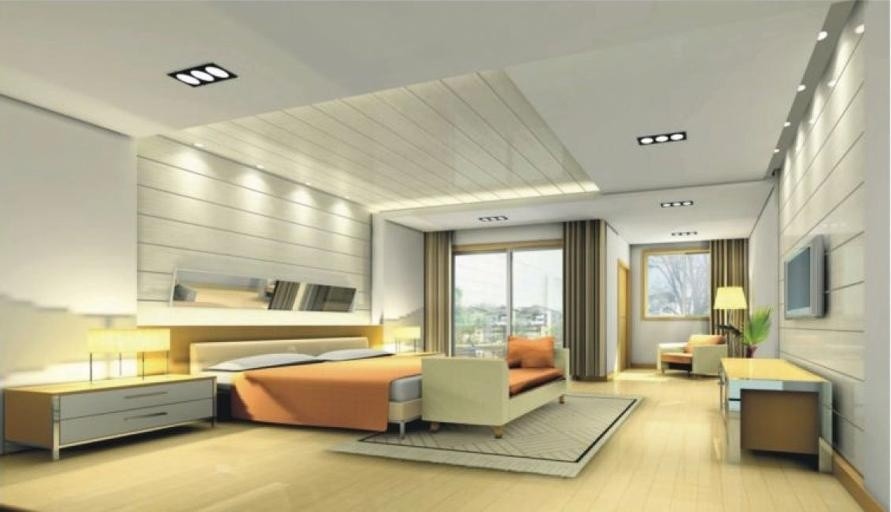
[421, 347, 570, 439]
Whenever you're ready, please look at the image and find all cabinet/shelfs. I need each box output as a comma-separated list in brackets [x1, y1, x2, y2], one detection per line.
[5, 372, 216, 461]
[717, 357, 833, 474]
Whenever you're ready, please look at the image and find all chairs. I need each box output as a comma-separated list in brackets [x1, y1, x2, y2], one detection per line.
[657, 341, 728, 380]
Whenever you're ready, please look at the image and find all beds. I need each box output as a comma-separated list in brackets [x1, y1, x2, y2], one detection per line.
[189, 337, 422, 439]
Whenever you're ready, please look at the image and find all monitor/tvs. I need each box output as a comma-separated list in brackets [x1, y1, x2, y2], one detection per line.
[783, 235, 825, 319]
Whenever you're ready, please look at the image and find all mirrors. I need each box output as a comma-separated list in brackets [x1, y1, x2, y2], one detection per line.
[169, 268, 268, 310]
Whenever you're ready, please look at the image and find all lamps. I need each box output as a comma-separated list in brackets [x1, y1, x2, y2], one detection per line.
[401, 325, 421, 352]
[84, 326, 171, 382]
[712, 286, 747, 335]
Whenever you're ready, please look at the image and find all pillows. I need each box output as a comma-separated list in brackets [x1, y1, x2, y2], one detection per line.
[202, 353, 325, 371]
[508, 335, 556, 367]
[318, 347, 396, 362]
[686, 334, 723, 354]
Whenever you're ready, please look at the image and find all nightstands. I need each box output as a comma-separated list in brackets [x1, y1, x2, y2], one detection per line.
[393, 351, 439, 357]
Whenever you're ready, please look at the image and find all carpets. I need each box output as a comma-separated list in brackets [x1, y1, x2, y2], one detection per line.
[323, 386, 644, 478]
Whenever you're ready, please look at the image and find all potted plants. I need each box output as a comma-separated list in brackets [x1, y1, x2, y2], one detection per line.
[735, 302, 775, 358]
